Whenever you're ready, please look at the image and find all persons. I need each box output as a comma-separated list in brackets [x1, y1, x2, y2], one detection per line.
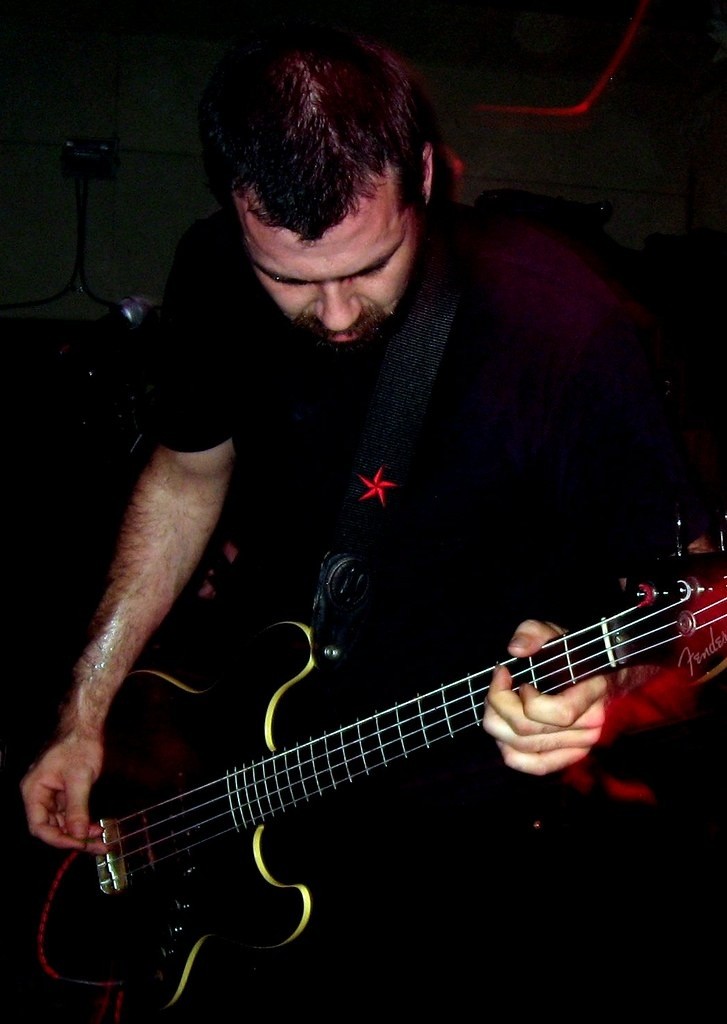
[19, 47, 726, 1024]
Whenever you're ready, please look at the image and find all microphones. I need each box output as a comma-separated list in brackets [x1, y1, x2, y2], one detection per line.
[57, 297, 150, 355]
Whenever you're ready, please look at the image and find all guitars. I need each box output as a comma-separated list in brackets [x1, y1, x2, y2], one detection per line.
[36, 550, 727, 1013]
